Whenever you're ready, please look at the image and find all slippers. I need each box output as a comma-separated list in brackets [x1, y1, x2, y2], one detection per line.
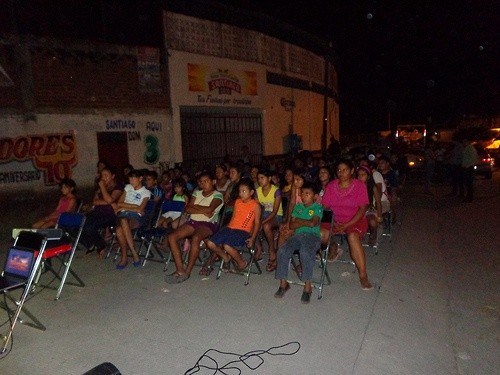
[222, 259, 231, 272]
[250, 255, 264, 265]
[165, 272, 189, 283]
[266, 259, 277, 271]
[199, 266, 214, 276]
[233, 262, 249, 273]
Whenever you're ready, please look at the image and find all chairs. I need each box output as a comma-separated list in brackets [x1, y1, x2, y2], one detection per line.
[0, 182, 400, 359]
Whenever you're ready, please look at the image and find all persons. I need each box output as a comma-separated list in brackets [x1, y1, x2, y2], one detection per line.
[206, 178, 262, 274]
[32, 178, 77, 229]
[166, 171, 224, 282]
[322, 159, 372, 288]
[116, 170, 152, 270]
[275, 182, 324, 302]
[452, 139, 474, 193]
[255, 170, 284, 272]
[78, 165, 124, 259]
[71, 138, 408, 249]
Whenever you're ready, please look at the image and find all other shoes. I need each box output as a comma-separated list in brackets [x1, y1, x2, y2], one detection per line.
[98, 247, 107, 258]
[134, 259, 140, 267]
[368, 235, 375, 246]
[85, 246, 96, 257]
[301, 288, 312, 304]
[116, 261, 128, 270]
[328, 247, 341, 263]
[276, 282, 290, 297]
[359, 275, 372, 291]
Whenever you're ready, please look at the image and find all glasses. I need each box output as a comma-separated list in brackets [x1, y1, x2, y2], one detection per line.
[336, 169, 349, 173]
